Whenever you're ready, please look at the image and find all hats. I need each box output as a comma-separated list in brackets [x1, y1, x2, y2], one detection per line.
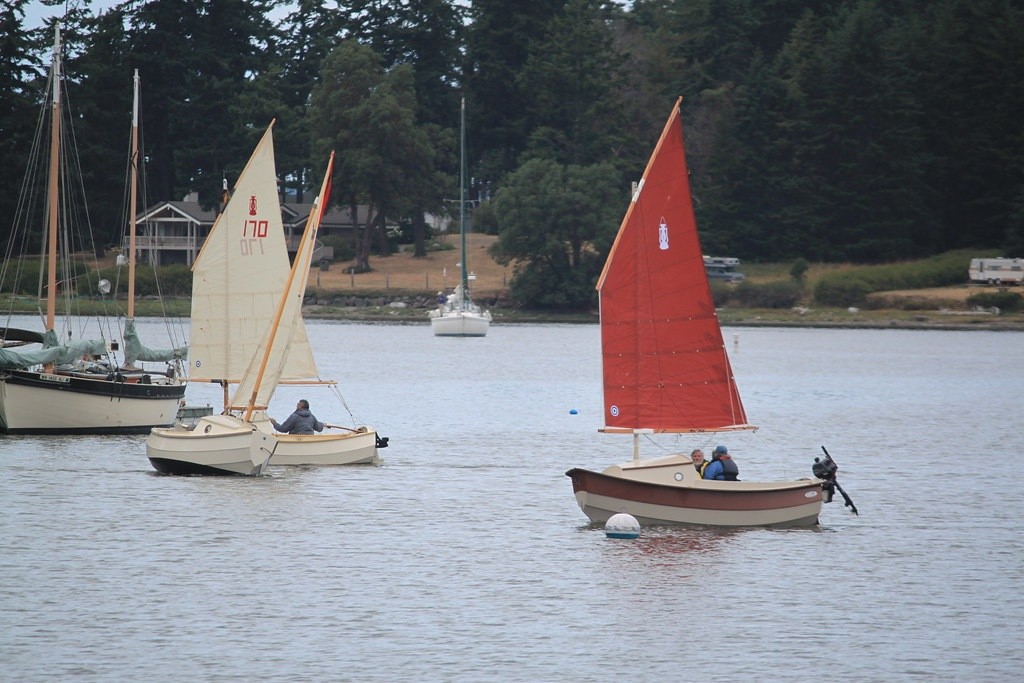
[712, 446, 728, 456]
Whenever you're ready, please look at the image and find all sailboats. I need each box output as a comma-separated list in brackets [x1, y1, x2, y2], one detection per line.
[426, 97, 494, 336]
[142, 146, 337, 479]
[175, 117, 392, 466]
[0, 19, 215, 439]
[560, 93, 861, 530]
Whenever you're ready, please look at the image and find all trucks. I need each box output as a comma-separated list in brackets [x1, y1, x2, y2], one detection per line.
[703, 255, 746, 284]
[968, 256, 1024, 286]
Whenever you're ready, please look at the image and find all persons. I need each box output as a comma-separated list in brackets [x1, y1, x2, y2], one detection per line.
[691, 446, 738, 481]
[436, 292, 447, 317]
[269, 400, 324, 435]
